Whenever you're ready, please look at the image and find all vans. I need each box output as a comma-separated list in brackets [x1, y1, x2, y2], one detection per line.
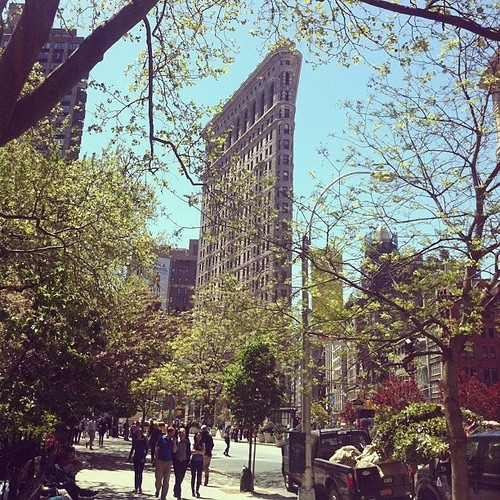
[410, 431, 499, 499]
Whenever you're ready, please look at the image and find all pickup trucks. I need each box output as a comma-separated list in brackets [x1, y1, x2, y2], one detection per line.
[274, 427, 408, 498]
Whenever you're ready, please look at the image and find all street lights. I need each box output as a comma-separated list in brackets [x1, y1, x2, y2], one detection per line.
[296, 169, 399, 499]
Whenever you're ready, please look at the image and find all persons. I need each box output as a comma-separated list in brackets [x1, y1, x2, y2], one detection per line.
[173, 428, 191, 500]
[154, 427, 177, 500]
[222, 425, 231, 457]
[40, 416, 250, 500]
[128, 430, 148, 494]
[190, 432, 205, 498]
